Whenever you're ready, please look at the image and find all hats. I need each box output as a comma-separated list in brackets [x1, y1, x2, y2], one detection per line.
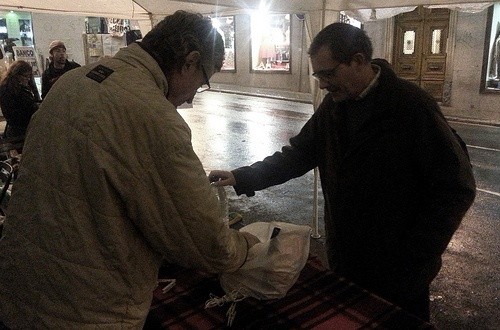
[48, 40, 67, 54]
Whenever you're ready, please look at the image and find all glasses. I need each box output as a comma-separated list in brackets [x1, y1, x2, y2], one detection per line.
[312, 61, 340, 79]
[197, 62, 211, 92]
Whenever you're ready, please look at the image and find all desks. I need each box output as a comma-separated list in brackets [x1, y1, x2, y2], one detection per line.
[146, 220, 440, 330]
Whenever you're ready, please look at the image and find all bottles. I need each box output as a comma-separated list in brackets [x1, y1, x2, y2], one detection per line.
[210, 177, 229, 230]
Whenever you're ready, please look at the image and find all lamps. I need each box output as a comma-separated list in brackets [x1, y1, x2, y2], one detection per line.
[369, 9, 378, 20]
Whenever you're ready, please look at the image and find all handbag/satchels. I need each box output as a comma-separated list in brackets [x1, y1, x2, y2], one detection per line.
[220, 221, 312, 303]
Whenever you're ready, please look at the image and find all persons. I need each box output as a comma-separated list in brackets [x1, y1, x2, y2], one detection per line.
[208, 23, 476, 318]
[0, 60, 38, 152]
[41, 40, 81, 100]
[0, 8, 263, 330]
